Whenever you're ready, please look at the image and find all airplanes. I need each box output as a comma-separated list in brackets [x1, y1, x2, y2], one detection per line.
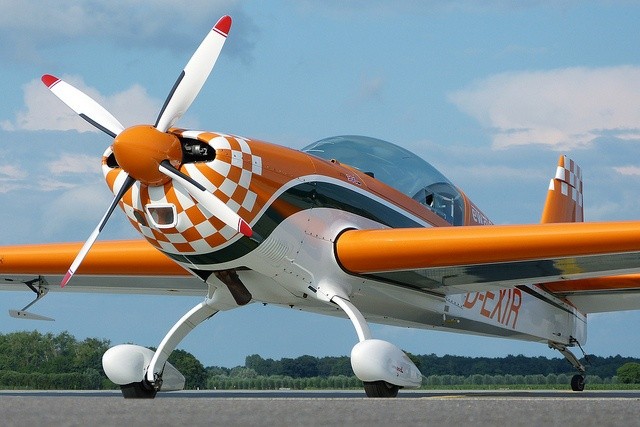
[1, 15, 636, 397]
